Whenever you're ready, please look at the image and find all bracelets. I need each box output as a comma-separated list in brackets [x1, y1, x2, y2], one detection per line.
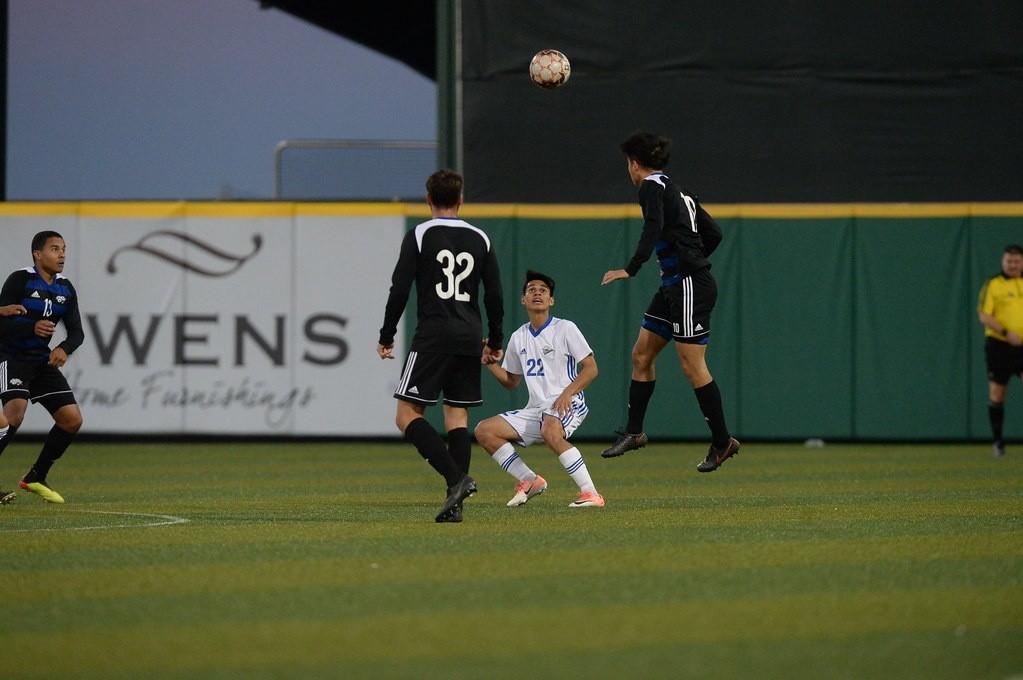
[1000, 328, 1008, 337]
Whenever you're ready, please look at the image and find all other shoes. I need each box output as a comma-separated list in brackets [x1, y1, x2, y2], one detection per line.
[994, 443, 1005, 457]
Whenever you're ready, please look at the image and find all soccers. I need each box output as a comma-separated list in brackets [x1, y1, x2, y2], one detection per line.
[529, 49, 571, 92]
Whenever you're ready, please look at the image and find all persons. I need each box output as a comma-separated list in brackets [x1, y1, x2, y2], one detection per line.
[0, 230, 85, 511]
[600, 132, 741, 473]
[376, 170, 506, 522]
[474, 270, 606, 506]
[977, 245, 1023, 457]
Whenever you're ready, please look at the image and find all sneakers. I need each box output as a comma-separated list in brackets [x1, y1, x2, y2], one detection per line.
[569, 492, 605, 508]
[435, 473, 478, 523]
[600, 427, 648, 458]
[0, 490, 17, 505]
[506, 474, 547, 508]
[697, 436, 740, 473]
[19, 473, 64, 504]
